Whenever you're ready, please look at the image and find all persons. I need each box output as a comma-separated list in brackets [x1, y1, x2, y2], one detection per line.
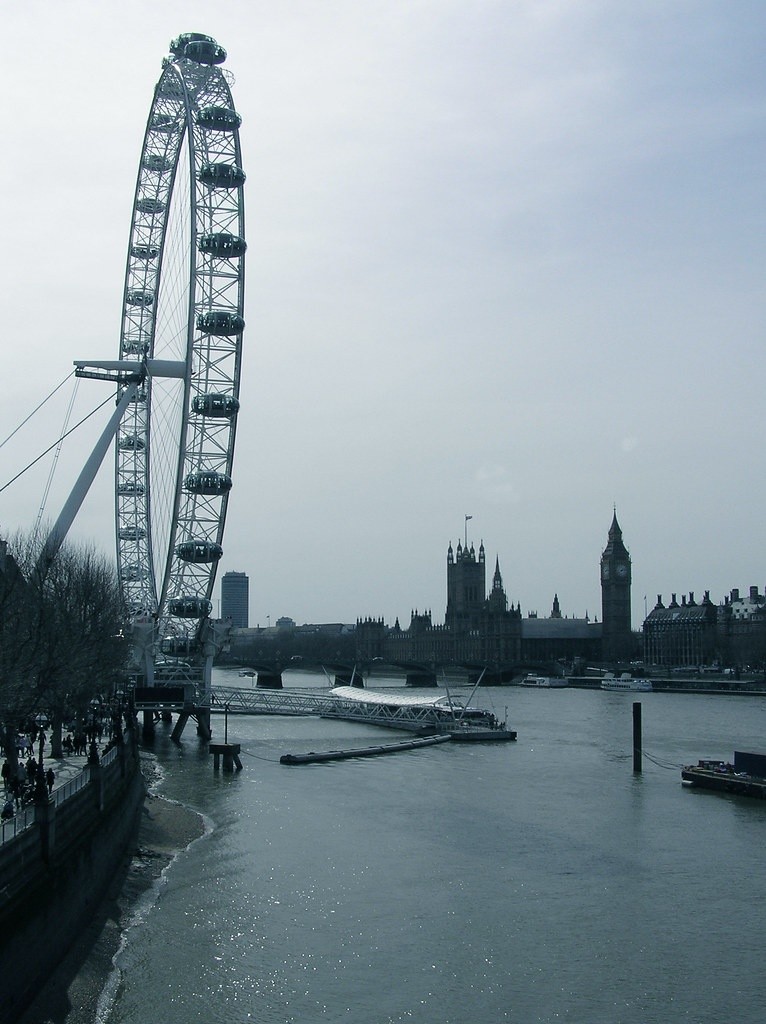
[0, 718, 124, 826]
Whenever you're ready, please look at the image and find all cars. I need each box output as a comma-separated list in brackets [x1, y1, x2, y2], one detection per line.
[292, 656, 302, 660]
[373, 657, 384, 660]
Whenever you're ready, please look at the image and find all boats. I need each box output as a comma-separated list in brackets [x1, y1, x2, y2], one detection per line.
[239, 671, 255, 678]
[519, 673, 570, 688]
[600, 677, 654, 692]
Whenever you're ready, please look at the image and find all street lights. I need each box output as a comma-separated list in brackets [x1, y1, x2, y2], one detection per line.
[33, 711, 52, 802]
[86, 696, 102, 769]
[113, 689, 124, 742]
